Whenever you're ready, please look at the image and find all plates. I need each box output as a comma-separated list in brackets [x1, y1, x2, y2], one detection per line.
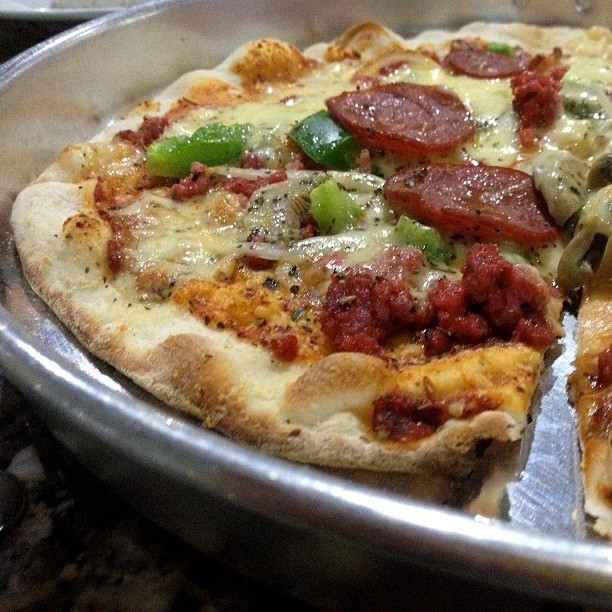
[2, 0, 611, 609]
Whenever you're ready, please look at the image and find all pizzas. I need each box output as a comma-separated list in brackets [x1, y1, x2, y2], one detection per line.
[9, 20, 611, 541]
